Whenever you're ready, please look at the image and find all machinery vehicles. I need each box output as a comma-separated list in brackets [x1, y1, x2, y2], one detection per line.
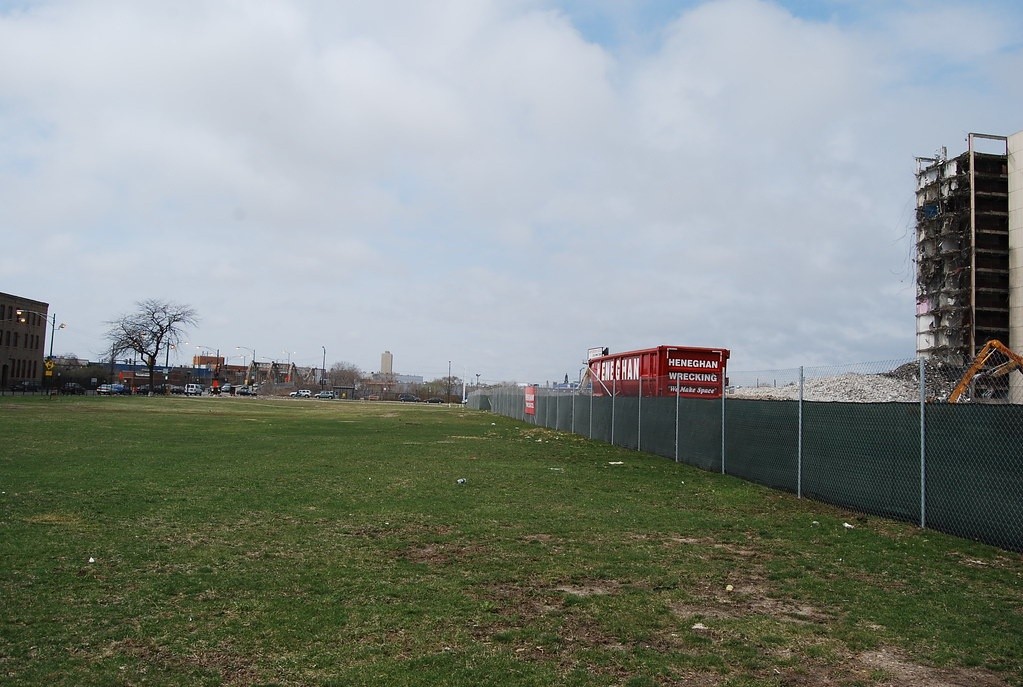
[925, 338, 1023, 426]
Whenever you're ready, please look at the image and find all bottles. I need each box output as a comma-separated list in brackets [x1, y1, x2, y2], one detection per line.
[456, 478, 466, 484]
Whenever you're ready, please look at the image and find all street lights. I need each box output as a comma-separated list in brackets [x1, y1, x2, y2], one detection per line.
[161, 340, 296, 371]
[448, 360, 452, 404]
[322, 345, 326, 390]
[17, 310, 66, 399]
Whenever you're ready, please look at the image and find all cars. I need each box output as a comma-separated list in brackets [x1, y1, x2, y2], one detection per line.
[61, 382, 86, 394]
[112, 385, 132, 395]
[134, 380, 266, 396]
[10, 381, 38, 392]
[96, 384, 113, 395]
[424, 398, 445, 404]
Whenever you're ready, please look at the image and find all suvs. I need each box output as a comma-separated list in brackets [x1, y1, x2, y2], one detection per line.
[397, 393, 421, 403]
[314, 391, 333, 399]
[290, 390, 311, 398]
